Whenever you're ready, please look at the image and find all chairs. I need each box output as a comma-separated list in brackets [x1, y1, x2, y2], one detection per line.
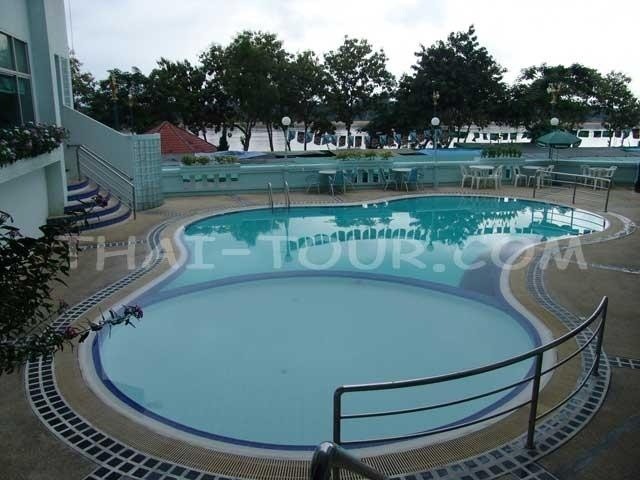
[581, 163, 617, 190]
[458, 162, 555, 191]
[380, 166, 425, 192]
[299, 164, 359, 195]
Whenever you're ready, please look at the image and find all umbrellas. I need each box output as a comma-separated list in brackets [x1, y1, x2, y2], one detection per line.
[533, 130, 582, 184]
[531, 207, 569, 242]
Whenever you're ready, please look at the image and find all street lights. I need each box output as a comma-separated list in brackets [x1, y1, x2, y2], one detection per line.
[282, 116, 292, 168]
[430, 117, 442, 191]
[550, 117, 560, 160]
